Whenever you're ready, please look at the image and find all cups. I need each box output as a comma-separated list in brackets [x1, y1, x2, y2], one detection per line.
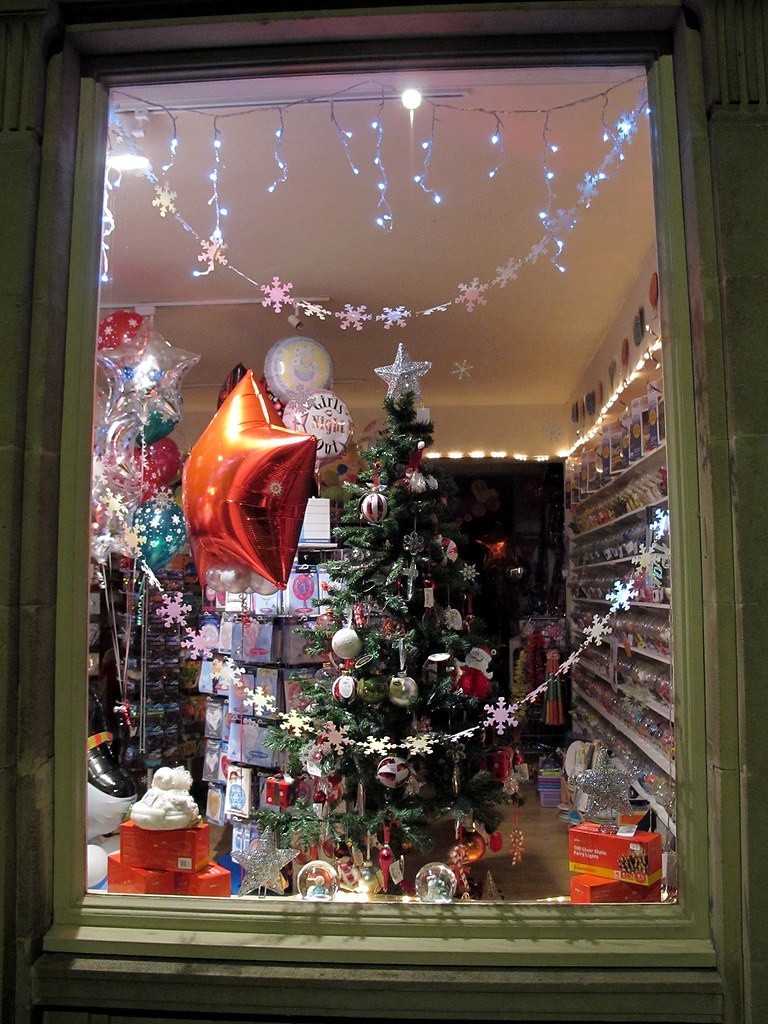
[653, 587, 663, 603]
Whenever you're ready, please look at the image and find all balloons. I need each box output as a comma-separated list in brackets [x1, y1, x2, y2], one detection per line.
[93, 391, 148, 567]
[137, 392, 184, 445]
[282, 388, 353, 459]
[96, 313, 202, 423]
[264, 334, 334, 406]
[132, 500, 187, 575]
[179, 363, 317, 590]
[133, 436, 181, 503]
[98, 310, 148, 369]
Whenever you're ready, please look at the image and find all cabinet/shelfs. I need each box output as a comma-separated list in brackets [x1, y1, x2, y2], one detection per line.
[109, 559, 200, 786]
[564, 399, 677, 844]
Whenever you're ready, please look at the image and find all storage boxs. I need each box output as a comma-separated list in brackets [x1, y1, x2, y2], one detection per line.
[569, 874, 661, 904]
[568, 821, 662, 888]
[107, 855, 174, 895]
[264, 779, 289, 807]
[118, 820, 211, 874]
[174, 862, 231, 897]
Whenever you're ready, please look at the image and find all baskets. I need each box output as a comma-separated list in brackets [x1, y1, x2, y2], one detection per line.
[537, 756, 562, 808]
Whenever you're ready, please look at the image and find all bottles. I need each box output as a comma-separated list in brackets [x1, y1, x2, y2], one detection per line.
[569, 467, 666, 602]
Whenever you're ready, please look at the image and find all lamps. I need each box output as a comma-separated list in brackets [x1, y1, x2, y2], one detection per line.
[106, 122, 149, 169]
